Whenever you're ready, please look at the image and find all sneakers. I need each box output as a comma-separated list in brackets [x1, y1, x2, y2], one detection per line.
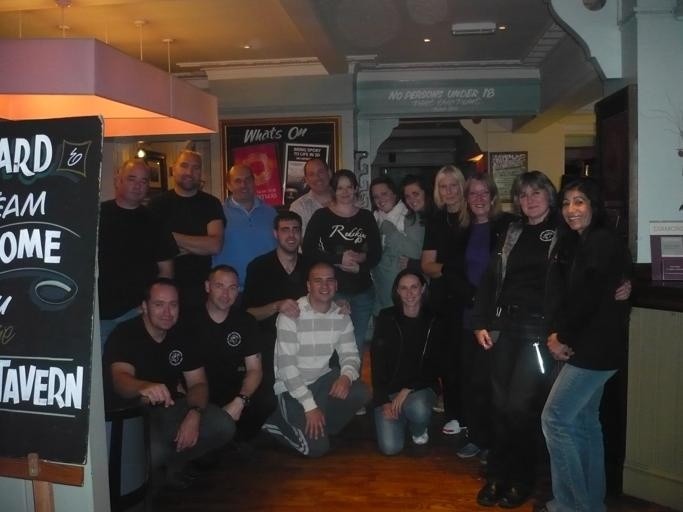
[455, 442, 479, 459]
[412, 430, 428, 444]
[442, 419, 462, 434]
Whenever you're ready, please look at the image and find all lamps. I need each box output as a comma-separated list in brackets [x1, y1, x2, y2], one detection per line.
[0, 0, 219, 134]
[463, 143, 484, 175]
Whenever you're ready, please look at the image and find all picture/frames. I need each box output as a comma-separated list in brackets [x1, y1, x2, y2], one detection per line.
[490, 151, 530, 203]
[140, 150, 168, 193]
[220, 117, 344, 211]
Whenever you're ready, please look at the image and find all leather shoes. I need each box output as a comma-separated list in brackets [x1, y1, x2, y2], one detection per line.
[499, 484, 524, 509]
[478, 482, 498, 505]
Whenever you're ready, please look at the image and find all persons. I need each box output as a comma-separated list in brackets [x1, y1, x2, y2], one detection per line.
[98, 158, 172, 343]
[442, 172, 506, 435]
[540, 179, 627, 512]
[363, 176, 425, 349]
[103, 280, 236, 480]
[145, 150, 226, 317]
[243, 211, 353, 410]
[212, 164, 278, 292]
[302, 169, 383, 415]
[288, 158, 335, 252]
[253, 260, 371, 458]
[420, 164, 466, 412]
[464, 171, 631, 512]
[400, 173, 434, 217]
[371, 267, 449, 456]
[183, 265, 277, 438]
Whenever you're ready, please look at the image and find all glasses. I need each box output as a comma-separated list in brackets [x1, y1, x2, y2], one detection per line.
[311, 279, 337, 287]
[469, 189, 490, 198]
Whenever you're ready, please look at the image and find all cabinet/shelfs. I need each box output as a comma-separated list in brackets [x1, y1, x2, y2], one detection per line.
[620, 271, 683, 508]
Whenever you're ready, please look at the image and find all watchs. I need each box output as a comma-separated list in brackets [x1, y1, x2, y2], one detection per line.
[189, 406, 203, 417]
[237, 393, 251, 404]
